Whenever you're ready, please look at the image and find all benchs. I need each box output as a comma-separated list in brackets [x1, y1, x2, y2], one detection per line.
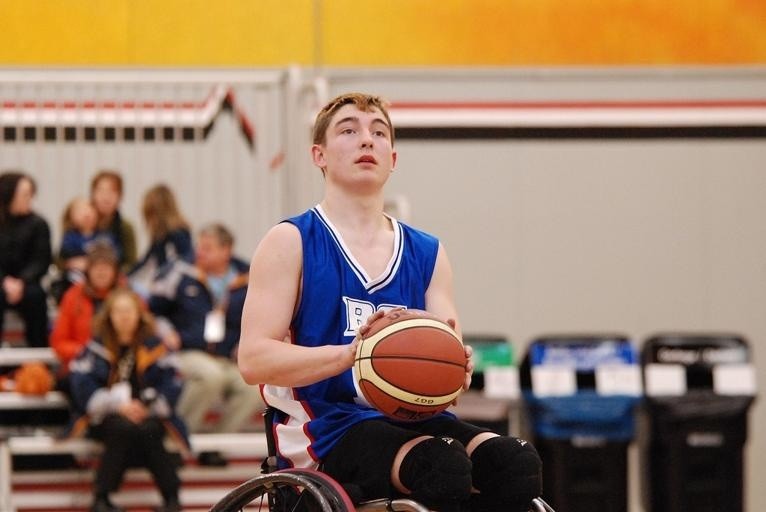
[5, 434, 268, 512]
[0, 247, 292, 512]
[1, 346, 73, 425]
[2, 299, 60, 341]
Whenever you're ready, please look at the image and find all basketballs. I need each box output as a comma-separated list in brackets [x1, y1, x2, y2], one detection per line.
[354, 309, 468, 424]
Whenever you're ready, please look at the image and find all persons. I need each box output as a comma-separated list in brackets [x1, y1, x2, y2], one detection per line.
[236, 93, 548, 512]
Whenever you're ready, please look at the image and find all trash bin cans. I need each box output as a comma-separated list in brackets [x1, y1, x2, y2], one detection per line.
[459, 336, 518, 437]
[638, 332, 758, 512]
[518, 333, 645, 511]
